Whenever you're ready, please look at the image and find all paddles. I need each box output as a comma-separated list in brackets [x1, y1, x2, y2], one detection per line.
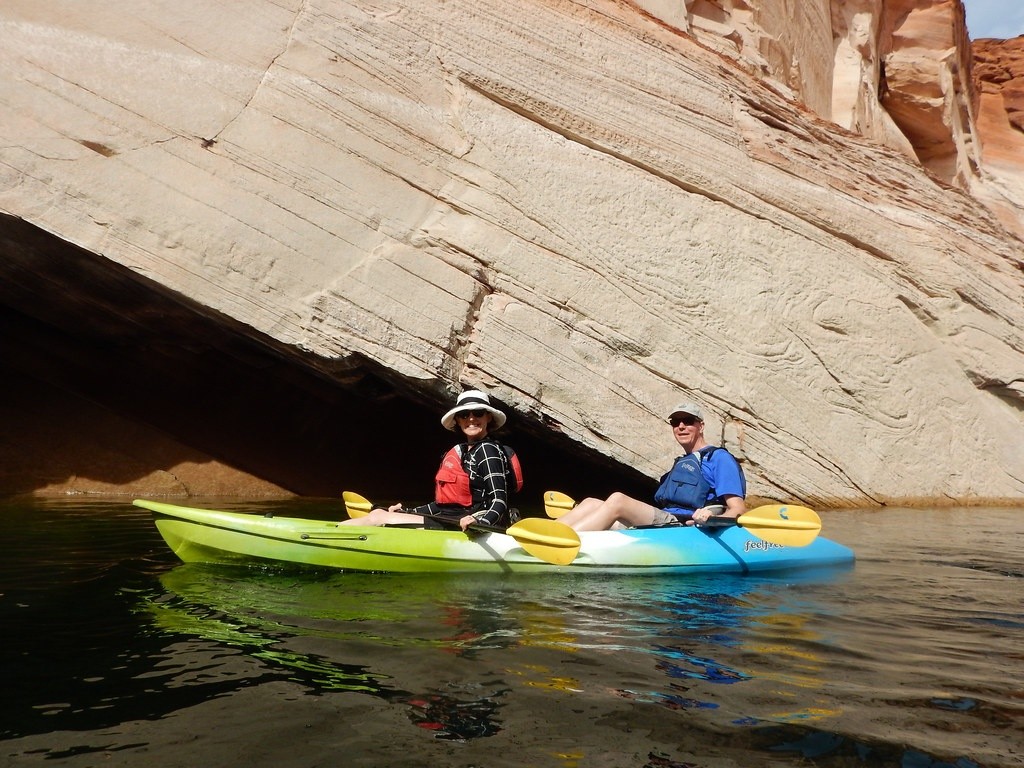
[542, 490, 823, 549]
[341, 490, 581, 568]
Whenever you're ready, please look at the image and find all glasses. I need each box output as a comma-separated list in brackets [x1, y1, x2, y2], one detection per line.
[670, 417, 696, 427]
[456, 409, 487, 419]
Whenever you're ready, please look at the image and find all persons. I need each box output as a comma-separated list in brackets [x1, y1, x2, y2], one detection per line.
[333, 387, 511, 534]
[551, 402, 747, 533]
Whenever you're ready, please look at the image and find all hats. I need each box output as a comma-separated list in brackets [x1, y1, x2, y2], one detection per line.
[668, 403, 704, 423]
[441, 390, 507, 433]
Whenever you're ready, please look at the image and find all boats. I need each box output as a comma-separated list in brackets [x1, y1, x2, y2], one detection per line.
[131, 489, 858, 582]
